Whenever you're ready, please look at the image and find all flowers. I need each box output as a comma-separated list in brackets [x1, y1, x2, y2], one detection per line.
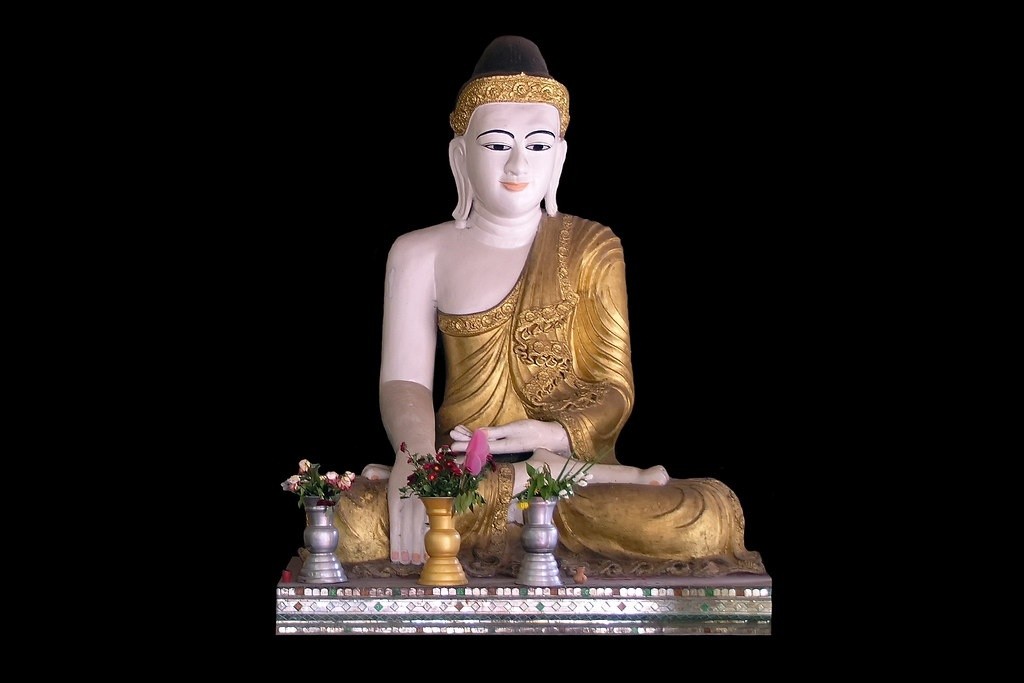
[398, 430, 496, 515]
[514, 448, 615, 509]
[281, 460, 355, 515]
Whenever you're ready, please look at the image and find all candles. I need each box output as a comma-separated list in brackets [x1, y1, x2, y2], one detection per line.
[282, 568, 292, 582]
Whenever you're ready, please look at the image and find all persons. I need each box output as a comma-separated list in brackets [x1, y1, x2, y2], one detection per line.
[327, 33, 745, 563]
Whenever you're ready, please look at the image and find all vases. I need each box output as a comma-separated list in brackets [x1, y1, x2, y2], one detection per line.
[416, 497, 468, 586]
[514, 497, 562, 587]
[297, 495, 350, 584]
